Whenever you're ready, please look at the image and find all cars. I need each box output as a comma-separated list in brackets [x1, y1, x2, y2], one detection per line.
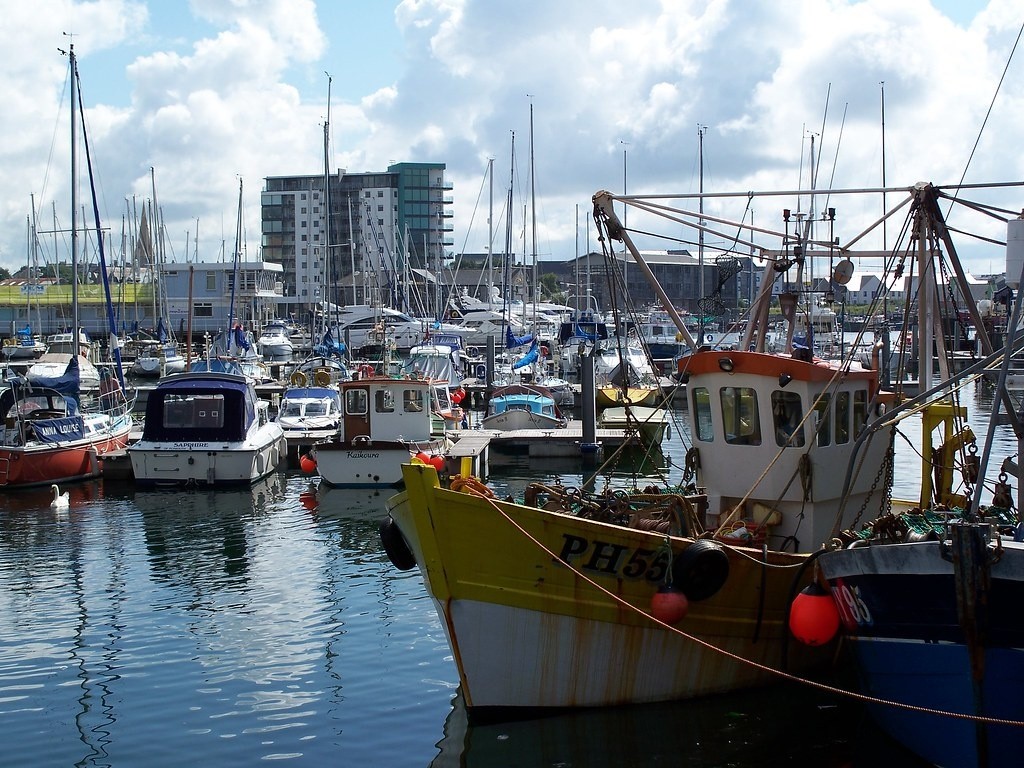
[742, 299, 750, 306]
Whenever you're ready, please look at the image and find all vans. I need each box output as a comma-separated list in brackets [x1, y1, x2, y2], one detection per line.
[820, 297, 836, 306]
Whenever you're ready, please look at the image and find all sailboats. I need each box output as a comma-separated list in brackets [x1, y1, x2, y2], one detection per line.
[0, 42, 910, 490]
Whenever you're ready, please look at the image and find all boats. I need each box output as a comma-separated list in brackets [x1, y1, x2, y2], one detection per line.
[310, 371, 446, 488]
[302, 480, 402, 525]
[384, 179, 1024, 727]
[125, 370, 287, 487]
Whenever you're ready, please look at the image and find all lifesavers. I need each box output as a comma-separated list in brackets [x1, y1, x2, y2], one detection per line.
[451, 311, 458, 317]
[315, 371, 330, 387]
[379, 517, 416, 570]
[670, 539, 732, 601]
[291, 370, 307, 386]
[707, 334, 713, 342]
[357, 364, 374, 380]
[233, 323, 244, 330]
